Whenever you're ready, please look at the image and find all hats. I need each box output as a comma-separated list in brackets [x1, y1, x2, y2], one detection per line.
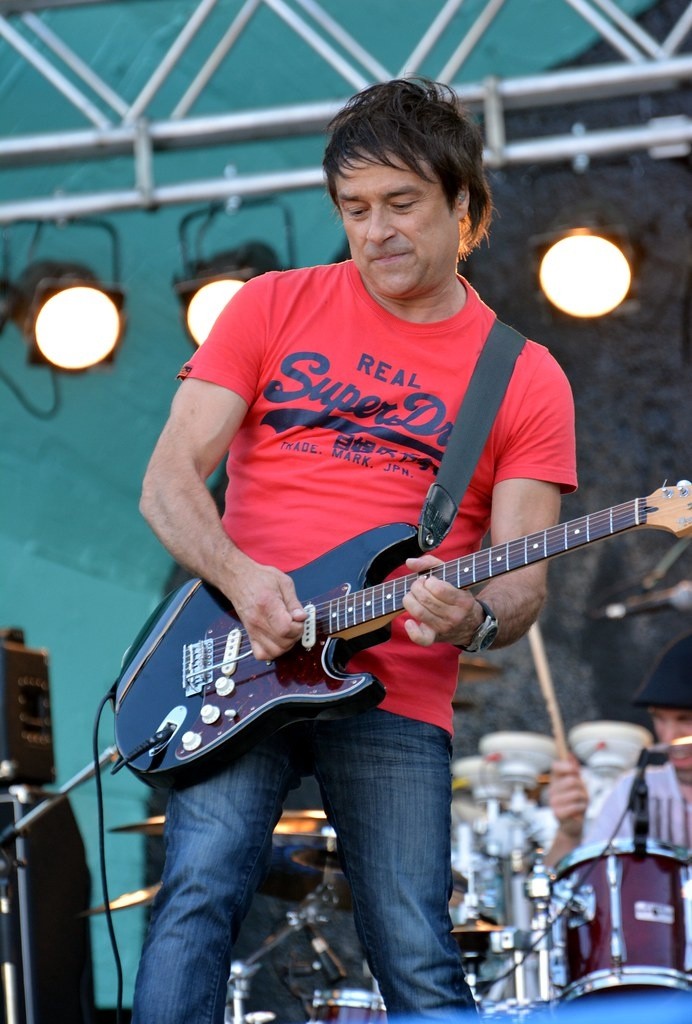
[631, 633, 692, 713]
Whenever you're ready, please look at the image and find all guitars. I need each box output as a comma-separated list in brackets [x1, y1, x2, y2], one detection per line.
[113, 479, 692, 793]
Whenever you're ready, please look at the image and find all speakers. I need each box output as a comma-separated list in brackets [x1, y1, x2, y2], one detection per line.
[0, 787, 94, 1024]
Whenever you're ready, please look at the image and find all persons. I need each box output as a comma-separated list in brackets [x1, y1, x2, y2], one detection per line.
[131, 77, 578, 1024]
[544, 634, 692, 975]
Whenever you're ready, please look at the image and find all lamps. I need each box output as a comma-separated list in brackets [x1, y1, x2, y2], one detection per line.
[170, 195, 299, 348]
[0, 216, 128, 423]
[526, 194, 643, 320]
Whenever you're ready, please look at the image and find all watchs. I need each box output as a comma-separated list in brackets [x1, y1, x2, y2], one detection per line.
[452, 599, 499, 653]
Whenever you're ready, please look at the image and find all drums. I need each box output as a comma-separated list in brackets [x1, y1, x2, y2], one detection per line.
[309, 989, 387, 1024]
[548, 836, 692, 1010]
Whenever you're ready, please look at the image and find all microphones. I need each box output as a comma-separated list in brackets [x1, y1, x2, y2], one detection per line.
[632, 775, 651, 857]
[592, 578, 692, 621]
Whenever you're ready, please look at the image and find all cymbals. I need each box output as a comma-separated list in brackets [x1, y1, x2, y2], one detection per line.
[451, 699, 479, 715]
[450, 915, 507, 933]
[458, 659, 506, 685]
[106, 814, 166, 837]
[273, 810, 337, 838]
[73, 879, 161, 913]
[256, 838, 469, 915]
